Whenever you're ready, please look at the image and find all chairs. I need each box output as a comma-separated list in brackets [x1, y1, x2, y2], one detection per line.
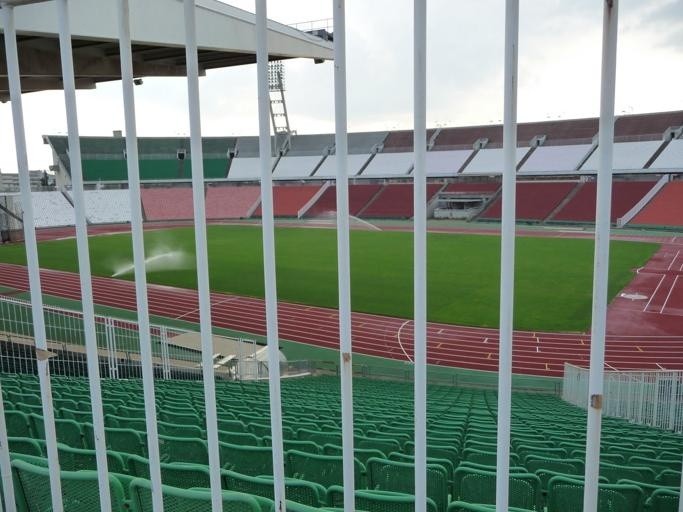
[0, 373, 683, 512]
